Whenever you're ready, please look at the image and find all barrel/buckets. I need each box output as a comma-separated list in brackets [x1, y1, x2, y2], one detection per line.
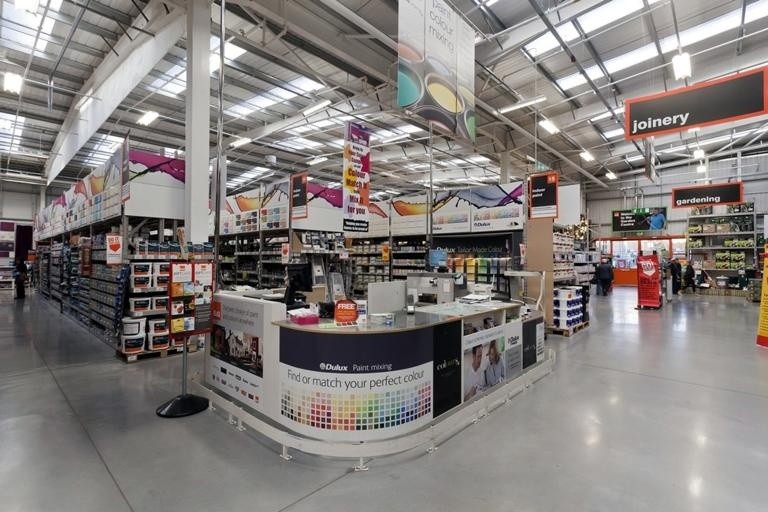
[552, 285, 582, 331]
[119, 260, 169, 355]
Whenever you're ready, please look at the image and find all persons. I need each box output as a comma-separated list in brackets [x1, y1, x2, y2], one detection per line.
[667, 257, 679, 295]
[680, 259, 697, 294]
[646, 207, 665, 230]
[594, 258, 614, 296]
[485, 339, 503, 388]
[463, 344, 487, 403]
[594, 261, 603, 295]
[14, 257, 28, 300]
[674, 256, 682, 291]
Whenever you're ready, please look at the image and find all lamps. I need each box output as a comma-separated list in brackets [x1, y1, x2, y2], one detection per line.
[697, 166, 705, 173]
[693, 149, 704, 161]
[671, 49, 693, 82]
[0, 0, 617, 182]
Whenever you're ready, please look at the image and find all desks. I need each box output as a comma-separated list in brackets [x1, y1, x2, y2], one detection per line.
[207, 270, 558, 460]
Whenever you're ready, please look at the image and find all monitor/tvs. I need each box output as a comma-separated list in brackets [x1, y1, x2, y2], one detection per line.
[284, 263, 313, 306]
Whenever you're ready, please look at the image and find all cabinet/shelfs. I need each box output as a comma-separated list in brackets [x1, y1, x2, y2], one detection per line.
[554, 226, 598, 336]
[343, 220, 554, 333]
[205, 228, 345, 309]
[685, 212, 768, 296]
[1, 223, 32, 292]
[31, 221, 215, 364]
